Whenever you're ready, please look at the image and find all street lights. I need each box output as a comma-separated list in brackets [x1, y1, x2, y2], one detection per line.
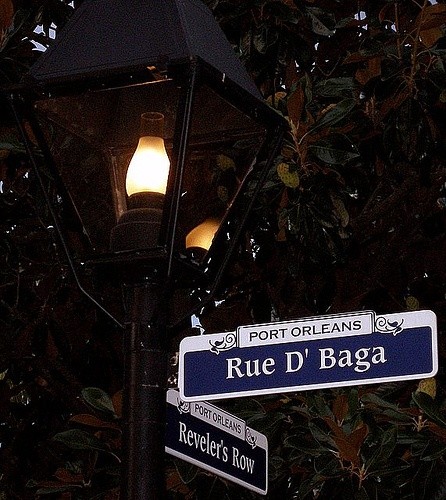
[7, 52, 292, 500]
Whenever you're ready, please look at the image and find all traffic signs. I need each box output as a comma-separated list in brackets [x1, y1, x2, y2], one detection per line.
[178, 309, 439, 403]
[165, 389, 269, 496]
